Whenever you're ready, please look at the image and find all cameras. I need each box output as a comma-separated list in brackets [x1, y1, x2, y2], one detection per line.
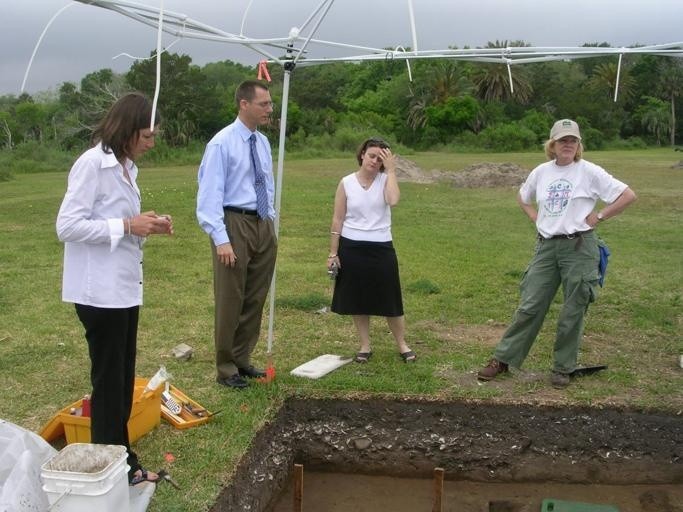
[328, 263, 339, 280]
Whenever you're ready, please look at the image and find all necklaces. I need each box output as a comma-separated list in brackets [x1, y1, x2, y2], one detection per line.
[357, 171, 376, 188]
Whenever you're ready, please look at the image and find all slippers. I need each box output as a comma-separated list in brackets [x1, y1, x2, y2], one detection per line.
[356, 353, 370, 362]
[400, 351, 417, 363]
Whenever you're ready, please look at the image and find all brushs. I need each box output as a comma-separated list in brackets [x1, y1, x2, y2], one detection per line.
[158, 470, 183, 490]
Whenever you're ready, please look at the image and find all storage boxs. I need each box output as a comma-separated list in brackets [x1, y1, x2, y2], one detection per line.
[39, 376, 165, 443]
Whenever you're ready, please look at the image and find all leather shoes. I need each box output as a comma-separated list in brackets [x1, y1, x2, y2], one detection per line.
[217, 366, 266, 387]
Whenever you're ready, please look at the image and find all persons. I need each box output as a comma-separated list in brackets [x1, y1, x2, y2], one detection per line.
[478, 119, 638, 389]
[327, 135, 417, 363]
[57, 91, 174, 483]
[195, 80, 279, 387]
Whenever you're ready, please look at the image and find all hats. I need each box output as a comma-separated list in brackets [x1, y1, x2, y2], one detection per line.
[550, 119, 582, 141]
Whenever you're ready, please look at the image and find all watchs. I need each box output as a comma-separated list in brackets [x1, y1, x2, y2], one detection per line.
[596, 210, 604, 222]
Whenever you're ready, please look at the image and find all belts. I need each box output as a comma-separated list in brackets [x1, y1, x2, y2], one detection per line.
[554, 230, 593, 249]
[223, 206, 257, 215]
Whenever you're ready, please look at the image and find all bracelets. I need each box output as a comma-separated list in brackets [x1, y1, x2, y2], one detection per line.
[126, 218, 131, 236]
[328, 254, 338, 258]
[331, 231, 340, 235]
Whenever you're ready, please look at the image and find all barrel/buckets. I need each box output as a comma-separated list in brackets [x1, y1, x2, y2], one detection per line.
[41, 443, 131, 512]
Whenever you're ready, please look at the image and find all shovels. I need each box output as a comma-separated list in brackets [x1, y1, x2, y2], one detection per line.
[191, 407, 224, 417]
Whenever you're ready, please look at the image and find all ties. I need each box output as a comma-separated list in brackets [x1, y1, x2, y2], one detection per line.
[250, 134, 268, 220]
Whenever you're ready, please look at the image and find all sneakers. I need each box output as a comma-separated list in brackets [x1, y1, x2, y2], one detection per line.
[552, 374, 569, 385]
[478, 359, 508, 380]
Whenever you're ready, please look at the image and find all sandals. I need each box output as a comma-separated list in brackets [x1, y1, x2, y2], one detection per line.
[134, 468, 159, 482]
